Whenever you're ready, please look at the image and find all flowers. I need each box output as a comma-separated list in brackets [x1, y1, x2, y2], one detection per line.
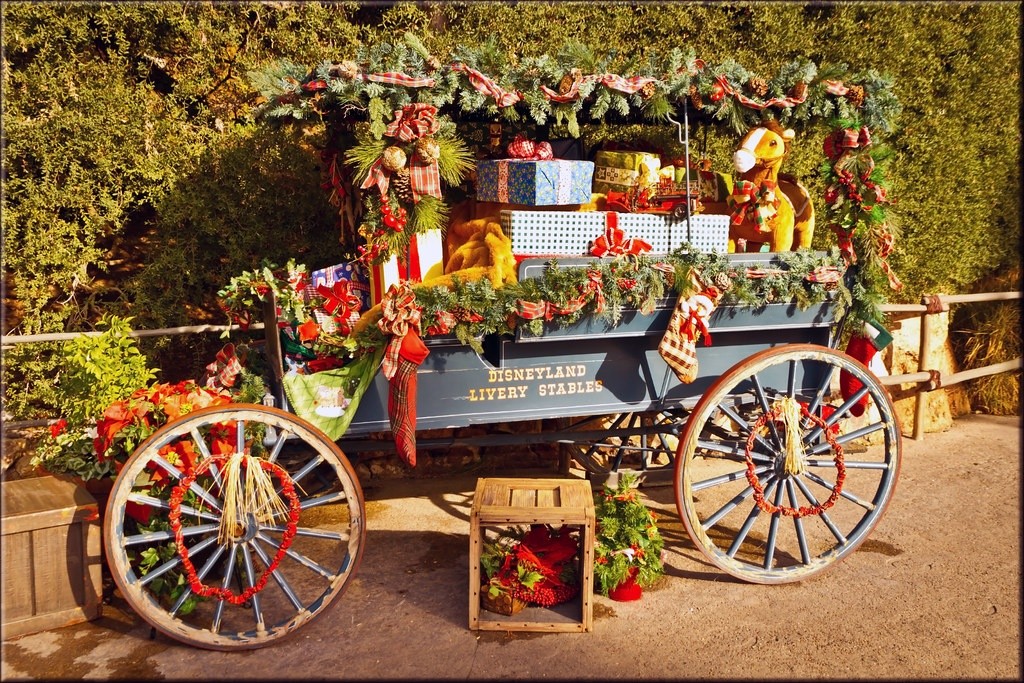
[593, 475, 665, 595]
[98, 379, 250, 473]
[480, 524, 580, 606]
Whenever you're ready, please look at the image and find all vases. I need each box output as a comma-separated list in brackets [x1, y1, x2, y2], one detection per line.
[609, 566, 640, 601]
[481, 581, 529, 616]
[125, 484, 223, 528]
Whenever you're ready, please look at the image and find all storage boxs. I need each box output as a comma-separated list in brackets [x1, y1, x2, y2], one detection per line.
[1, 473, 102, 640]
[307, 262, 367, 335]
[595, 150, 660, 196]
[474, 158, 595, 206]
